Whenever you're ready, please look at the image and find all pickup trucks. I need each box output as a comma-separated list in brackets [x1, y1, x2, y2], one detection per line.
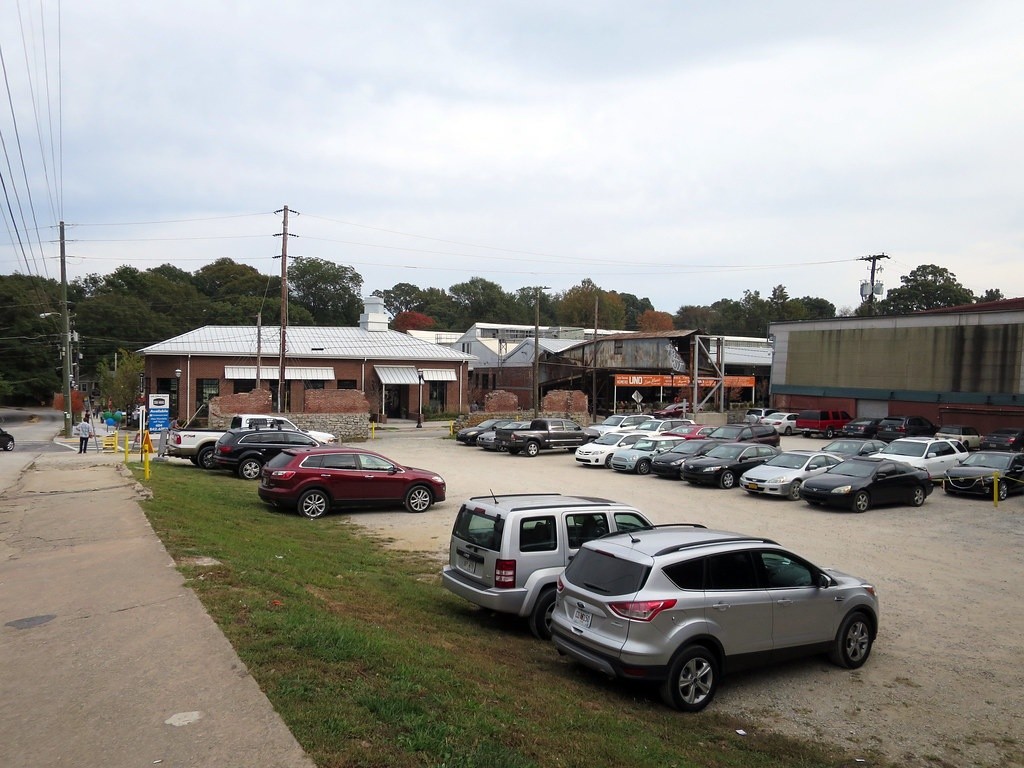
[494, 418, 601, 457]
[796, 410, 856, 438]
[162, 415, 311, 469]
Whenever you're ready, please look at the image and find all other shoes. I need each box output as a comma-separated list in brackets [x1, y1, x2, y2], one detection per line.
[77, 450, 87, 453]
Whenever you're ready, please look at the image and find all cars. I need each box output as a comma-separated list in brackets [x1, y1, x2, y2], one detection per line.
[0, 428, 14, 451]
[303, 430, 338, 447]
[575, 430, 933, 514]
[842, 417, 883, 439]
[820, 428, 1024, 514]
[654, 402, 704, 418]
[760, 412, 802, 434]
[456, 417, 532, 452]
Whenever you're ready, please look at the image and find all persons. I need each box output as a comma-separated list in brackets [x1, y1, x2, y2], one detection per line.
[85, 410, 90, 423]
[76, 418, 91, 454]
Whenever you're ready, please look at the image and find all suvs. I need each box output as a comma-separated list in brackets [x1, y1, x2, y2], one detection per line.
[588, 415, 780, 447]
[442, 493, 879, 712]
[877, 415, 938, 443]
[744, 408, 778, 425]
[211, 427, 325, 480]
[257, 446, 447, 519]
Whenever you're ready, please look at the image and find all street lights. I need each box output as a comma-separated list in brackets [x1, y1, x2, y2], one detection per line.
[40, 312, 73, 438]
[175, 368, 182, 419]
[416, 369, 424, 428]
